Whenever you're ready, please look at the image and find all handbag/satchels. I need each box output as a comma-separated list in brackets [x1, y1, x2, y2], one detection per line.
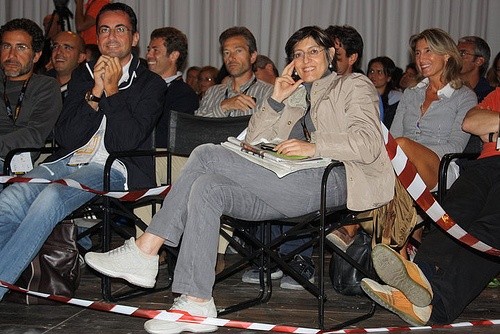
[330, 232, 381, 296]
[6, 223, 81, 306]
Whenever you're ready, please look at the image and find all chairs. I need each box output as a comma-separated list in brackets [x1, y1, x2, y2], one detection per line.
[3, 85, 496, 333]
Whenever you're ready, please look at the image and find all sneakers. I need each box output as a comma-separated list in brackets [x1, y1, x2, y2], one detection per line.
[372, 243, 434, 308]
[85, 236, 160, 289]
[280, 255, 315, 290]
[144, 296, 219, 334]
[243, 262, 283, 284]
[360, 276, 433, 327]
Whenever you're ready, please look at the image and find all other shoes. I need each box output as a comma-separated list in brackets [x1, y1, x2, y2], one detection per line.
[325, 222, 360, 252]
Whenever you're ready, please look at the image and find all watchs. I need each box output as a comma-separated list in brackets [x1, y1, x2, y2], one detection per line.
[84, 91, 102, 103]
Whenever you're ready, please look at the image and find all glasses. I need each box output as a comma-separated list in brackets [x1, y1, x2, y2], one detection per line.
[198, 76, 213, 82]
[3, 43, 29, 52]
[462, 52, 472, 57]
[99, 26, 133, 37]
[290, 48, 324, 60]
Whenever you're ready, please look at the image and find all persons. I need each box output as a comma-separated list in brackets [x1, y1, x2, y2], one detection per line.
[0, 0, 500, 334]
